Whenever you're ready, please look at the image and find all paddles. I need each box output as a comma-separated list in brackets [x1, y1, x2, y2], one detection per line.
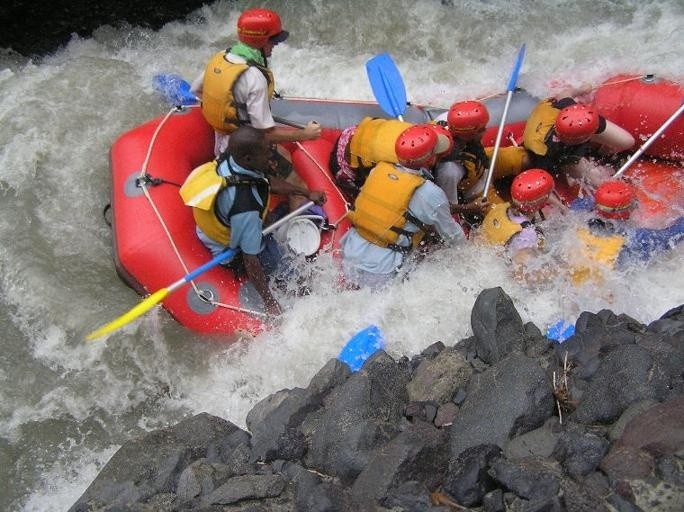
[153, 75, 308, 129]
[545, 214, 575, 341]
[338, 228, 431, 374]
[484, 46, 524, 200]
[85, 200, 316, 339]
[367, 53, 406, 123]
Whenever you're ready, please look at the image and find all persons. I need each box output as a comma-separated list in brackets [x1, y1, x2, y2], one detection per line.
[177, 127, 326, 327]
[200, 10, 324, 176]
[328, 78, 684, 312]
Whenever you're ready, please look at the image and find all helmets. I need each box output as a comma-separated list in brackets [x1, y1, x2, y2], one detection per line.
[395, 124, 453, 167]
[556, 104, 605, 145]
[237, 8, 289, 48]
[448, 101, 489, 137]
[596, 183, 634, 220]
[511, 169, 554, 213]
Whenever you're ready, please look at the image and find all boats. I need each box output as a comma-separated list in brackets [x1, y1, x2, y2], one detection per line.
[107, 74, 683, 343]
